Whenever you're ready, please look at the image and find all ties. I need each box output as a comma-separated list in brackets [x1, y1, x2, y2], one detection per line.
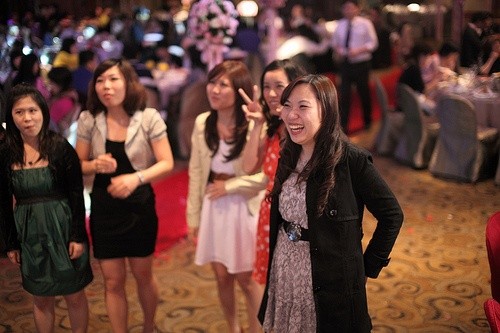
[344, 21, 352, 51]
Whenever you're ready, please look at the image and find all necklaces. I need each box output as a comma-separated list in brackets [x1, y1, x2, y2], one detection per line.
[300, 159, 308, 166]
[25, 155, 43, 167]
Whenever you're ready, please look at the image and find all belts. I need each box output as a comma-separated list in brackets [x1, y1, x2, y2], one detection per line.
[282, 221, 310, 242]
[207, 169, 235, 185]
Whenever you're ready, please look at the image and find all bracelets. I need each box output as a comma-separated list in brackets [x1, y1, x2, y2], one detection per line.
[137, 172, 145, 183]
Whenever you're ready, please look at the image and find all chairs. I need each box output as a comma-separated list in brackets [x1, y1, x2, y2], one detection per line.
[375, 78, 498, 184]
[172, 82, 211, 159]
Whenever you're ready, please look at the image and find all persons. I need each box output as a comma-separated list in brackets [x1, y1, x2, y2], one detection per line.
[256, 75, 404, 333]
[0, 84, 94, 333]
[0, 0, 500, 136]
[75, 58, 175, 333]
[187, 61, 270, 333]
[239, 59, 307, 277]
